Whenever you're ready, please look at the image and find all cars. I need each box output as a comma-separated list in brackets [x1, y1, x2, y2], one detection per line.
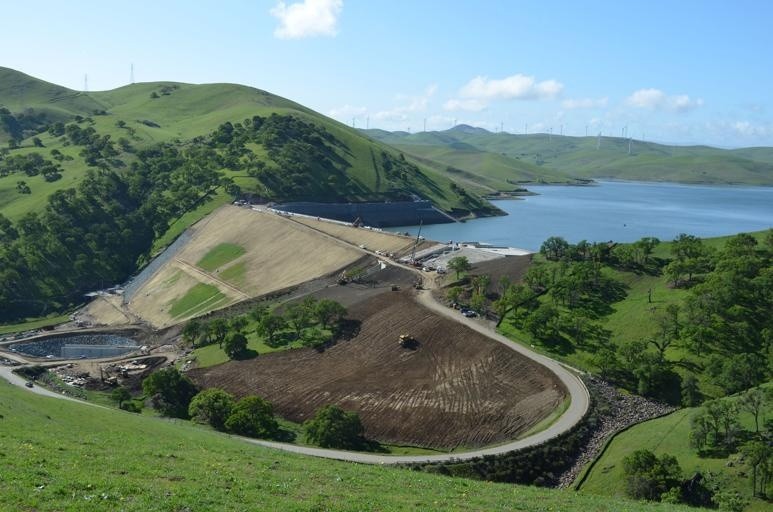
[359, 243, 478, 318]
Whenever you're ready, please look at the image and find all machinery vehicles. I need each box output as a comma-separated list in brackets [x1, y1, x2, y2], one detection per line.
[399, 334, 416, 347]
